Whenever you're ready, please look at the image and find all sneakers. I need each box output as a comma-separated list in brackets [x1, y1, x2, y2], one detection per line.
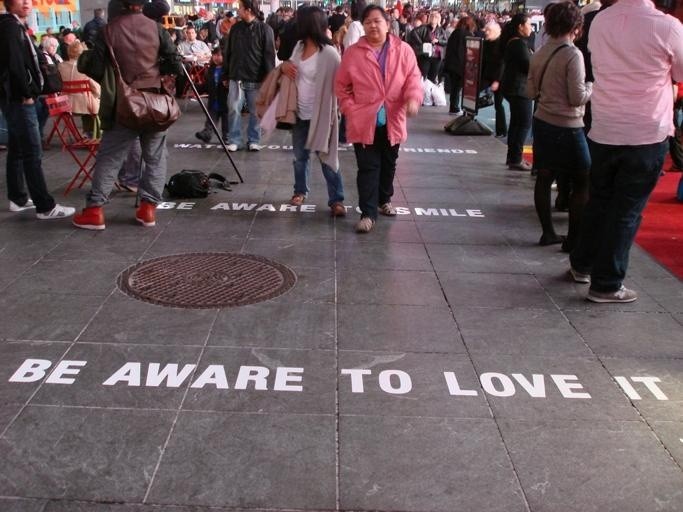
[506, 158, 530, 166]
[291, 192, 305, 206]
[508, 161, 531, 170]
[330, 202, 346, 217]
[195, 129, 260, 152]
[9, 199, 106, 229]
[586, 284, 638, 302]
[570, 266, 591, 282]
[357, 214, 376, 232]
[381, 202, 397, 215]
[135, 197, 157, 226]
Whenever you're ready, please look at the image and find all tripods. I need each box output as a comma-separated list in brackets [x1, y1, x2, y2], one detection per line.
[135, 37, 244, 209]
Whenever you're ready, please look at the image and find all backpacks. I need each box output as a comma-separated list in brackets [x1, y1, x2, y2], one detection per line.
[165, 169, 232, 198]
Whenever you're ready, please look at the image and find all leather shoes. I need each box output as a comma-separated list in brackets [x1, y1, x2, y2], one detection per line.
[539, 235, 566, 245]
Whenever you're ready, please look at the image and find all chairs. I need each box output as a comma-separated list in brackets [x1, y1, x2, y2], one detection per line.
[45, 95, 143, 195]
[182, 61, 210, 98]
[45, 79, 92, 150]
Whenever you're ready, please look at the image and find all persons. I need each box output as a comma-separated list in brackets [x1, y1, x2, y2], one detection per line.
[0, 0, 683, 234]
[568, 0, 682, 303]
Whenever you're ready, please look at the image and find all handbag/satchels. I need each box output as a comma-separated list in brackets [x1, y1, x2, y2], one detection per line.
[112, 74, 182, 130]
